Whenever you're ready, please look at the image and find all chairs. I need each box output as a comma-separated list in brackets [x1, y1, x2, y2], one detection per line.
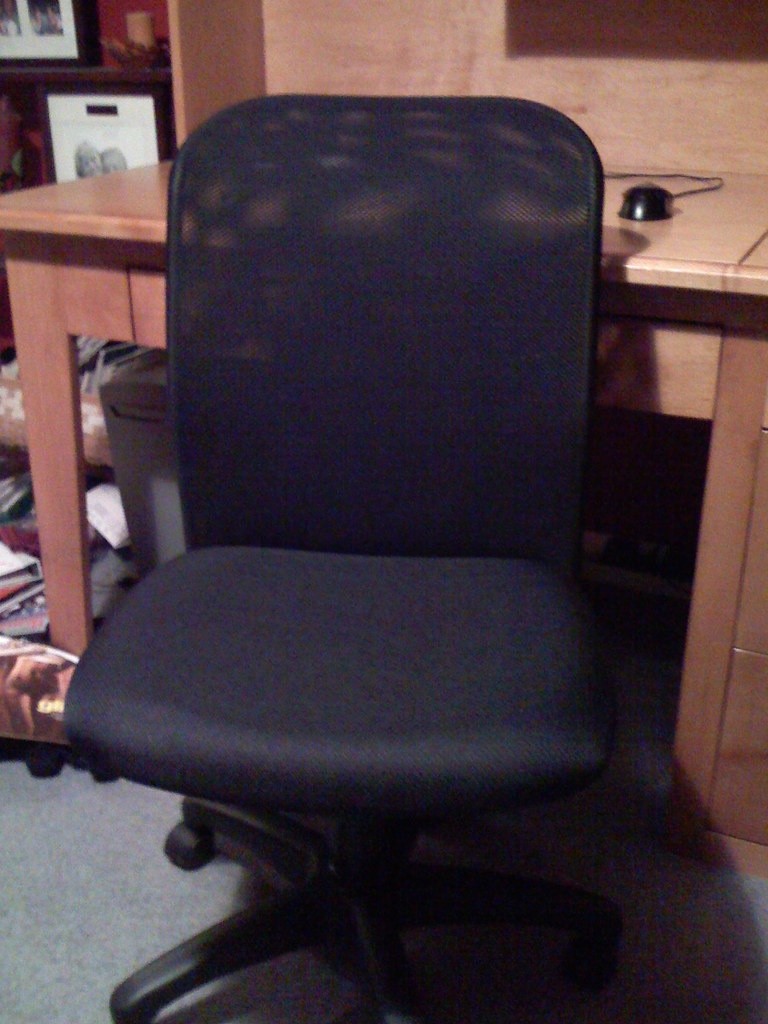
[62, 90, 625, 1024]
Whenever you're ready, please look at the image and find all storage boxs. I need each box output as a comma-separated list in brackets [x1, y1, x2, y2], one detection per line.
[43, 92, 171, 183]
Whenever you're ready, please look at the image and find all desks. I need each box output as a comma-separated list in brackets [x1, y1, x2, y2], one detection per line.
[0, 155, 768, 879]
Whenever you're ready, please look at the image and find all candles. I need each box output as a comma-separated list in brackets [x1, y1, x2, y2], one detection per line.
[125, 10, 156, 48]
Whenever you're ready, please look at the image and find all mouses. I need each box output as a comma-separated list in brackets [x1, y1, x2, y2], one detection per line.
[618, 182, 673, 223]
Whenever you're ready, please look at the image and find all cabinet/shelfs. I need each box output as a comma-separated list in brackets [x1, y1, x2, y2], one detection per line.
[0, 0, 267, 467]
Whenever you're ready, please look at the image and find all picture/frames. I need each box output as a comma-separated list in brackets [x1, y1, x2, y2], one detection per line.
[0, 0, 88, 62]
